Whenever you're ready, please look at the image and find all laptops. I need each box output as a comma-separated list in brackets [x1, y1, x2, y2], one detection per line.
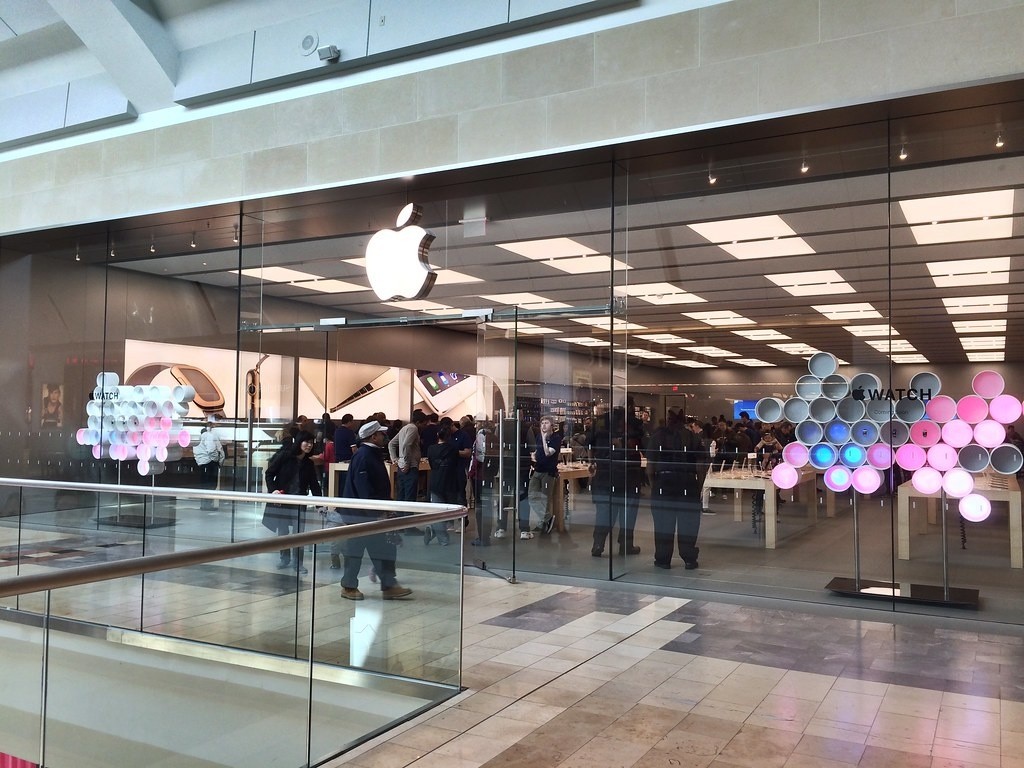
[299, 357, 395, 413]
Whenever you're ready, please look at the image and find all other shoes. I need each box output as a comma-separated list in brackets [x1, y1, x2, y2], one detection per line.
[294, 563, 308, 574]
[368, 569, 377, 582]
[653, 559, 672, 569]
[620, 545, 640, 555]
[439, 538, 448, 546]
[404, 528, 424, 535]
[276, 559, 291, 568]
[455, 526, 466, 533]
[702, 507, 717, 515]
[590, 543, 604, 556]
[445, 524, 456, 531]
[200, 506, 218, 511]
[684, 559, 698, 569]
[330, 559, 340, 569]
[423, 527, 432, 544]
[471, 538, 482, 547]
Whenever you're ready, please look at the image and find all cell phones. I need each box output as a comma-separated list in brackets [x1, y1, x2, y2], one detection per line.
[414, 370, 478, 416]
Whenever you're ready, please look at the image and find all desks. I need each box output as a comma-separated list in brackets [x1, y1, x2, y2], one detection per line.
[554, 463, 591, 532]
[329, 459, 431, 513]
[213, 459, 268, 511]
[703, 463, 836, 549]
[898, 464, 1023, 568]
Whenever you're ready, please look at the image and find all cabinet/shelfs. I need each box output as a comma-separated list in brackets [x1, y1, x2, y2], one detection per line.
[634, 405, 653, 422]
[515, 399, 596, 445]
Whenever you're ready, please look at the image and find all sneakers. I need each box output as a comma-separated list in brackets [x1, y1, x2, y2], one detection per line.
[521, 531, 534, 539]
[382, 581, 412, 599]
[341, 587, 363, 600]
[543, 515, 556, 534]
[532, 522, 545, 533]
[494, 529, 506, 537]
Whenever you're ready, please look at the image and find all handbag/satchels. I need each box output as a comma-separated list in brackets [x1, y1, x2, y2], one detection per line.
[479, 456, 498, 475]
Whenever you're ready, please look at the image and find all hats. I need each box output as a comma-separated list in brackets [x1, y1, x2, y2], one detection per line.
[359, 421, 387, 439]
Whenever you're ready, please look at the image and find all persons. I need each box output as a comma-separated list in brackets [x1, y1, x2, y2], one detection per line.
[42, 384, 63, 428]
[321, 508, 348, 570]
[261, 431, 326, 575]
[283, 408, 534, 547]
[191, 429, 226, 511]
[585, 395, 645, 557]
[467, 420, 495, 546]
[555, 411, 798, 523]
[424, 423, 459, 546]
[646, 406, 706, 570]
[340, 422, 413, 600]
[527, 416, 561, 534]
[388, 412, 426, 536]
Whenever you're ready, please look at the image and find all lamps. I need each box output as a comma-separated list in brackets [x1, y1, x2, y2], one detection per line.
[900, 148, 908, 160]
[111, 250, 115, 256]
[802, 162, 808, 173]
[191, 236, 196, 248]
[150, 245, 155, 252]
[996, 137, 1003, 147]
[317, 45, 341, 61]
[233, 231, 239, 243]
[708, 174, 717, 184]
[76, 253, 80, 260]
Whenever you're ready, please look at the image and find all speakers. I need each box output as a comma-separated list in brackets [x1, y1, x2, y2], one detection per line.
[317, 45, 340, 62]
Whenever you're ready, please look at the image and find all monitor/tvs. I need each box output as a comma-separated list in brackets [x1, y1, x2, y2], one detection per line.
[733, 399, 760, 420]
